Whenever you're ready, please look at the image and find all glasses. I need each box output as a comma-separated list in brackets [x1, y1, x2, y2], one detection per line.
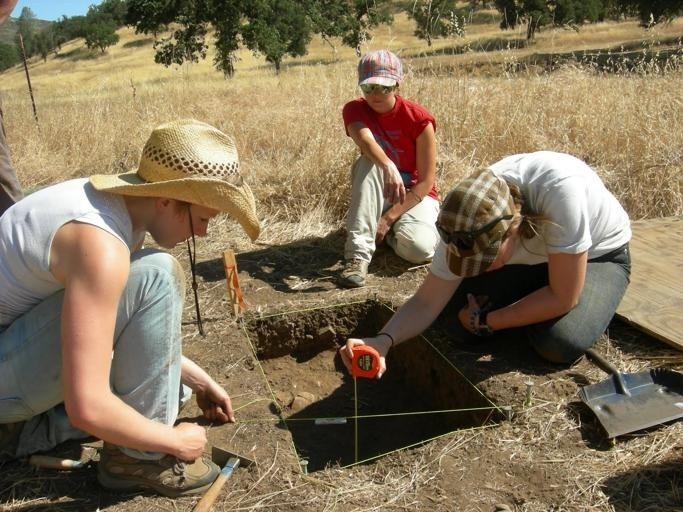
[359, 81, 395, 95]
[433, 214, 514, 252]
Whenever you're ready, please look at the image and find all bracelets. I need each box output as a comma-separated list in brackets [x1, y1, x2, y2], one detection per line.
[470, 310, 480, 339]
[376, 333, 394, 345]
[480, 313, 492, 337]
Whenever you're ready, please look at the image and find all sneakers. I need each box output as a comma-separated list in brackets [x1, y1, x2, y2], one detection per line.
[26, 441, 98, 470]
[95, 445, 223, 499]
[337, 257, 370, 289]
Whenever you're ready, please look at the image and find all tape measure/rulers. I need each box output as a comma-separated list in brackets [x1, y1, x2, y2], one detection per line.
[350, 344, 382, 464]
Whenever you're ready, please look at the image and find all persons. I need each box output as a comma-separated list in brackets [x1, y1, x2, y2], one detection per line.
[1, 118, 262, 498]
[340, 50, 440, 288]
[339, 151, 633, 381]
[1, 0, 23, 208]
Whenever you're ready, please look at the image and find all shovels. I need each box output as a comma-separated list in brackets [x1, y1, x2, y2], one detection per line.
[27, 442, 103, 483]
[577, 347, 683, 441]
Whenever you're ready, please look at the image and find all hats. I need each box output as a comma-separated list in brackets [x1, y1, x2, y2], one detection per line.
[433, 167, 516, 279]
[88, 118, 262, 244]
[357, 50, 404, 88]
